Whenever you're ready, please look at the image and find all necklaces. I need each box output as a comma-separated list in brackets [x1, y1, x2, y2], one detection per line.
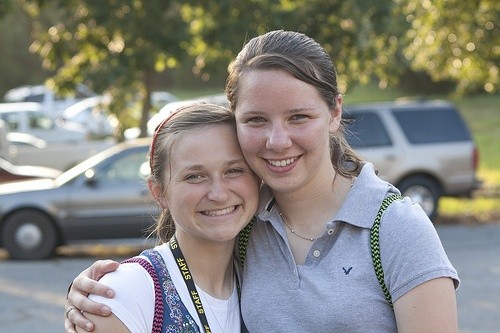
[271, 175, 355, 243]
[183, 242, 237, 333]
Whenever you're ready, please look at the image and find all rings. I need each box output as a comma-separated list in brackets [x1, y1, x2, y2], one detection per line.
[64, 308, 77, 320]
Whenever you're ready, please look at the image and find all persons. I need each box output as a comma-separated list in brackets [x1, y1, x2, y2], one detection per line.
[61, 31, 461, 333]
[66, 96, 263, 333]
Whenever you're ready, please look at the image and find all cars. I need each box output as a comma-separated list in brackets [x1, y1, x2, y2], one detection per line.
[0, 77, 234, 168]
[0, 139, 172, 261]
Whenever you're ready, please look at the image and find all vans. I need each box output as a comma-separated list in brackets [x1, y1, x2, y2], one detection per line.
[336, 99, 482, 224]
